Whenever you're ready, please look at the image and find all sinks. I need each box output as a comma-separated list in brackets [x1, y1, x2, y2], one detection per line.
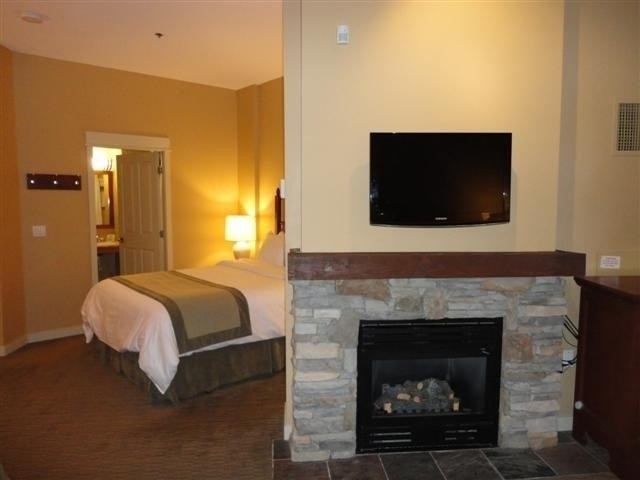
[97, 240, 119, 247]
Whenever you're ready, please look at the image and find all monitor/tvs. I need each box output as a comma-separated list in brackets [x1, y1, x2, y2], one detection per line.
[369, 131, 512, 227]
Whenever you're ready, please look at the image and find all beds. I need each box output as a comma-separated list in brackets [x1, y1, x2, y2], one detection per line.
[81, 188, 285, 405]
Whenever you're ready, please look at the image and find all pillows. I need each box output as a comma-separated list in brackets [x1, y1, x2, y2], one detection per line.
[258, 230, 285, 264]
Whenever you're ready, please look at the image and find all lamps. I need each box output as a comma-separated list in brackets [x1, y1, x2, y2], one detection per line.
[225, 215, 256, 260]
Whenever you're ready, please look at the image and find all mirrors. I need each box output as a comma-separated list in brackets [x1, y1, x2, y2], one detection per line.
[93, 170, 115, 228]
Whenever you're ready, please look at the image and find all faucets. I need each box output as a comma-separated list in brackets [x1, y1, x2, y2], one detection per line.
[96, 232, 99, 241]
